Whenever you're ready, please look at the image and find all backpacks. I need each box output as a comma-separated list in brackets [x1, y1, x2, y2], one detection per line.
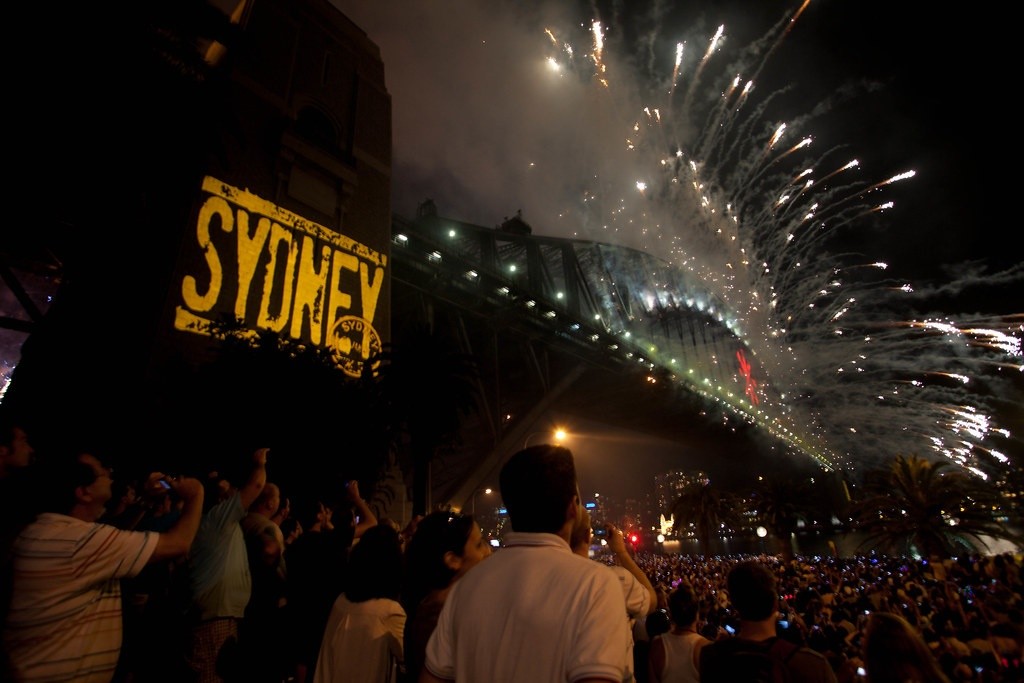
[714, 635, 802, 683]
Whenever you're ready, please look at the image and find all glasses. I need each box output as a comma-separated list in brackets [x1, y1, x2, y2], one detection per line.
[97, 467, 113, 477]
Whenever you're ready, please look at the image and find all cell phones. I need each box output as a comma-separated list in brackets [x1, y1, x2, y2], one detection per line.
[726, 625, 736, 635]
[159, 479, 171, 490]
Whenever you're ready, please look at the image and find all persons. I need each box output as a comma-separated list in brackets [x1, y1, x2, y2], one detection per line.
[0, 423, 492, 683]
[419, 444, 634, 683]
[572, 506, 1024, 683]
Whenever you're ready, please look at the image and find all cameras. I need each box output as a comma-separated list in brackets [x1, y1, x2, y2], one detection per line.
[776, 620, 790, 628]
[594, 527, 606, 536]
[489, 539, 500, 546]
[857, 666, 866, 676]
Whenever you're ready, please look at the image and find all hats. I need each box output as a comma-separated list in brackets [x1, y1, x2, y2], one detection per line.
[405, 510, 475, 590]
